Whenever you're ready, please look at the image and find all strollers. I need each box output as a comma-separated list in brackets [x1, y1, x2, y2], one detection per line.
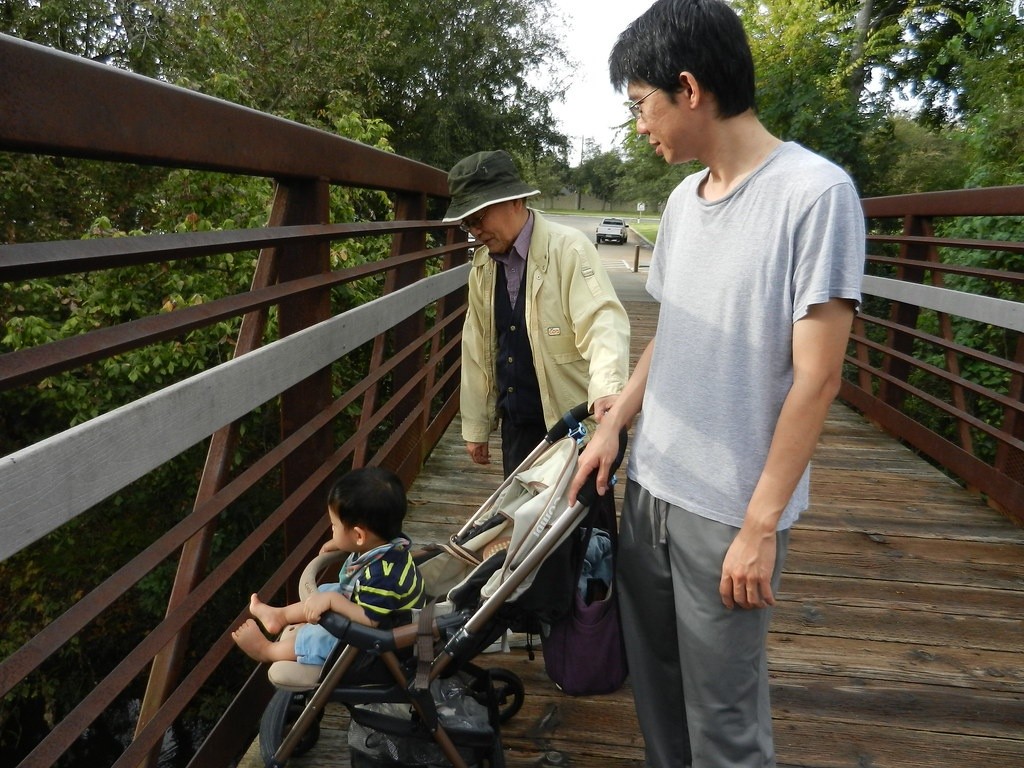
[258, 399, 631, 768]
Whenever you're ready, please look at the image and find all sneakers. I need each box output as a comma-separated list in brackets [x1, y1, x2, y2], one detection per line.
[508, 631, 542, 647]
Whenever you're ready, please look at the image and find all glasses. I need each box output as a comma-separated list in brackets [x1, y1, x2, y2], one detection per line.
[629, 83, 664, 117]
[459, 205, 491, 233]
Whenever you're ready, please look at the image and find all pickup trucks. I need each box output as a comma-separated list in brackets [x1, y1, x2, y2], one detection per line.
[596, 218, 630, 245]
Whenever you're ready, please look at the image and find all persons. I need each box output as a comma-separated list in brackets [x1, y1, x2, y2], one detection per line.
[232, 467, 426, 689]
[569, 0, 866, 768]
[443, 150, 631, 651]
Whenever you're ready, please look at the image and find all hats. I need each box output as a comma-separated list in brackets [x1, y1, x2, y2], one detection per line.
[442, 150, 541, 223]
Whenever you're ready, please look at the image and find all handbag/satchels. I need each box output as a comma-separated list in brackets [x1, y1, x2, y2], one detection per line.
[539, 480, 629, 695]
